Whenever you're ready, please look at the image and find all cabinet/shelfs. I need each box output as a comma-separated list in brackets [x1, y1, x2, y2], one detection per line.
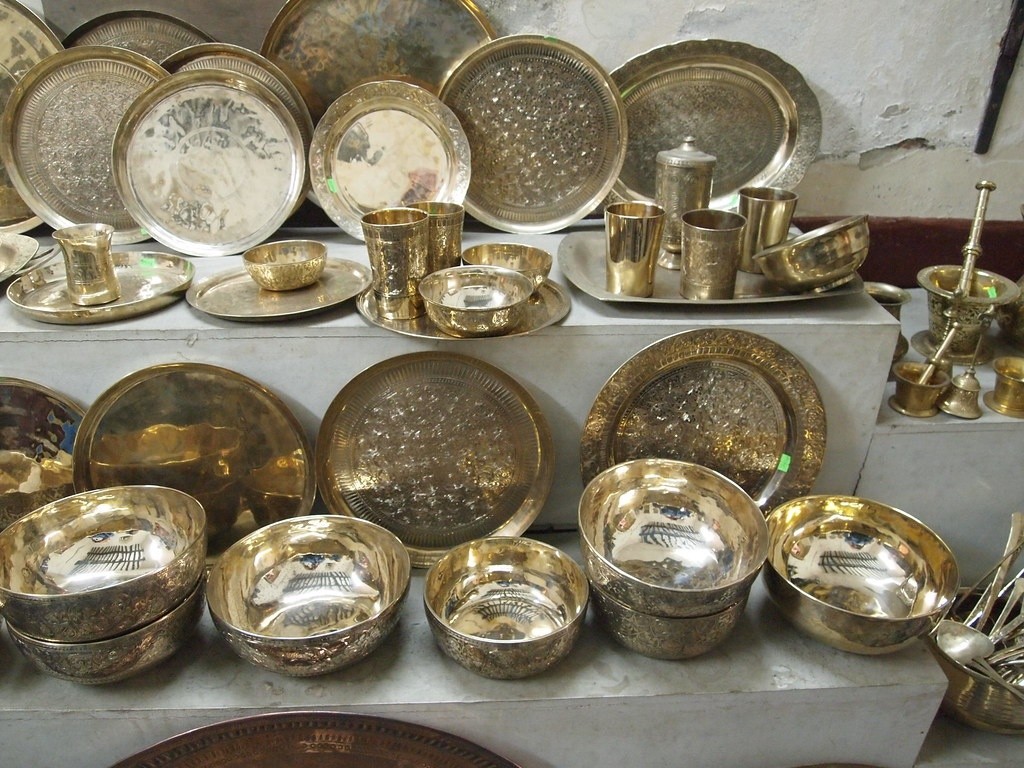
[0, 228, 1024, 768]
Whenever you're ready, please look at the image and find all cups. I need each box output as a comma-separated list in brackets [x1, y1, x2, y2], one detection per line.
[405, 202, 464, 271]
[49, 223, 122, 305]
[604, 201, 666, 298]
[738, 187, 797, 274]
[681, 208, 748, 300]
[361, 209, 430, 320]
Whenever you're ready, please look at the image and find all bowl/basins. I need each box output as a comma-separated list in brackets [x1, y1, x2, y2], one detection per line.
[241, 240, 329, 291]
[419, 241, 553, 338]
[0, 459, 958, 686]
[754, 214, 872, 293]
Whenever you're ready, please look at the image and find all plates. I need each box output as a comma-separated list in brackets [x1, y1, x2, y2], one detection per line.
[1, 232, 38, 281]
[1, 0, 821, 258]
[1, 329, 826, 572]
[559, 229, 759, 304]
[358, 280, 570, 340]
[6, 255, 196, 325]
[185, 257, 370, 323]
